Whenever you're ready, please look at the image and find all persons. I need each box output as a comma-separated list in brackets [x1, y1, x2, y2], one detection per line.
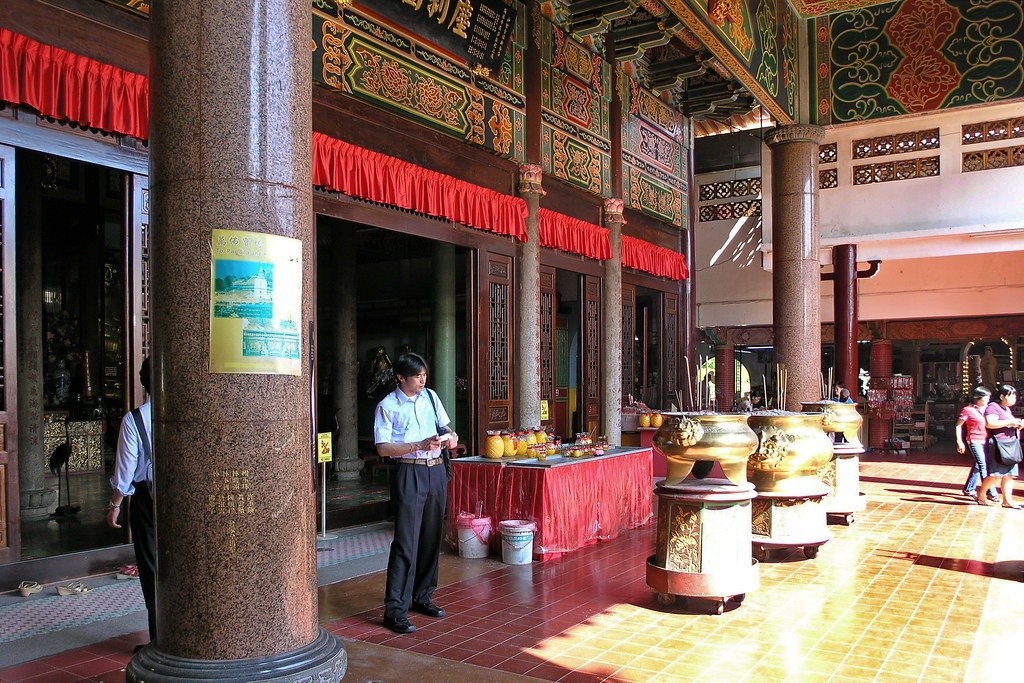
[702, 374, 716, 411]
[955, 385, 1024, 510]
[107, 356, 156, 653]
[831, 381, 853, 442]
[736, 392, 762, 412]
[374, 354, 459, 633]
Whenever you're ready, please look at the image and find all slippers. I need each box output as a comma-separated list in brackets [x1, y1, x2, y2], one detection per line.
[1002, 503, 1023, 509]
[972, 495, 989, 505]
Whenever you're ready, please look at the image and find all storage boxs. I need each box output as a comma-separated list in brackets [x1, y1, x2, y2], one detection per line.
[902, 442, 910, 448]
[1003, 370, 1013, 381]
[895, 442, 902, 449]
[909, 436, 924, 441]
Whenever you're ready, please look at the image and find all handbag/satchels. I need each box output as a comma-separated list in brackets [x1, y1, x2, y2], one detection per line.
[437, 426, 452, 481]
[995, 438, 1023, 466]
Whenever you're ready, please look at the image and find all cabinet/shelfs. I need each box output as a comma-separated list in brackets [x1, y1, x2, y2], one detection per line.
[893, 400, 932, 450]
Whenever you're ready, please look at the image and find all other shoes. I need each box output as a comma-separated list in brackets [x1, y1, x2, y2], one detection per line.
[987, 496, 1000, 502]
[962, 490, 977, 500]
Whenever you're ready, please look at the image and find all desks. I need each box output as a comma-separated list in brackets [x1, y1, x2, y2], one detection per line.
[448, 445, 655, 561]
[636, 427, 753, 482]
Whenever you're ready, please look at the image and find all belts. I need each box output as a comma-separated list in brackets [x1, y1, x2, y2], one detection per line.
[397, 458, 444, 466]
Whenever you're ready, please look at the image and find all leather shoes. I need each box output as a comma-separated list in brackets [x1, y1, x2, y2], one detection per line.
[412, 602, 446, 617]
[383, 618, 416, 633]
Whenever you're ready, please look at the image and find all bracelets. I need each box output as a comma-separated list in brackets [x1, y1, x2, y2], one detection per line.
[407, 443, 413, 452]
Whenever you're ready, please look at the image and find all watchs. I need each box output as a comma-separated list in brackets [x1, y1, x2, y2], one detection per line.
[108, 499, 120, 509]
